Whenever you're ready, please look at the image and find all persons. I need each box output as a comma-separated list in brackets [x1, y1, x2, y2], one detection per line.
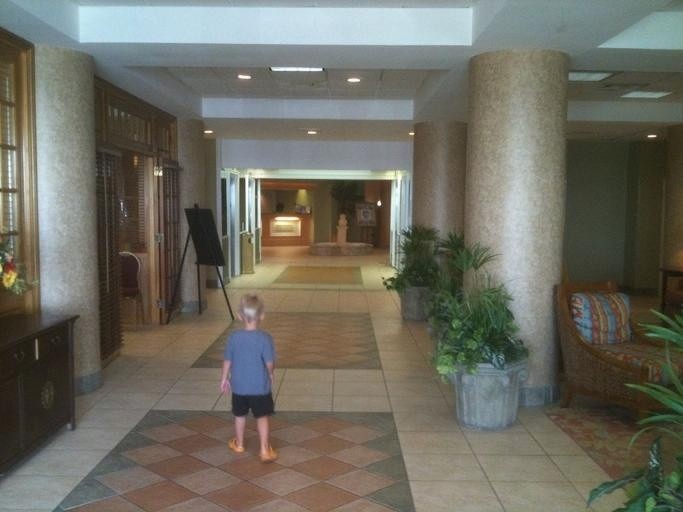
[219, 291, 281, 462]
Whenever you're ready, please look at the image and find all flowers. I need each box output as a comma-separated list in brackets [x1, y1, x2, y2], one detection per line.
[0, 224, 39, 294]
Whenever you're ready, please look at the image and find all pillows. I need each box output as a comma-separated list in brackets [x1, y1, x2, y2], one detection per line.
[571, 292, 633, 346]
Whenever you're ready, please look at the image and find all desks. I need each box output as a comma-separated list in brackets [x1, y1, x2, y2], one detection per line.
[659, 264, 683, 313]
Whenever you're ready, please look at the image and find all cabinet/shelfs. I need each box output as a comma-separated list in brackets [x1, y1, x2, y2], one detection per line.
[0, 311, 81, 481]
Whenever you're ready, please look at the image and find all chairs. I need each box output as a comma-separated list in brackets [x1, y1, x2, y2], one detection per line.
[117, 252, 145, 330]
[552, 279, 683, 424]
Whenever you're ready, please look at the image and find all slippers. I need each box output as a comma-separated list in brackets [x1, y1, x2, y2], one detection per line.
[261, 448, 279, 462]
[229, 438, 244, 452]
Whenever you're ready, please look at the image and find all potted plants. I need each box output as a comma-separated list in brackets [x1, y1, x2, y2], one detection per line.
[383, 224, 531, 432]
